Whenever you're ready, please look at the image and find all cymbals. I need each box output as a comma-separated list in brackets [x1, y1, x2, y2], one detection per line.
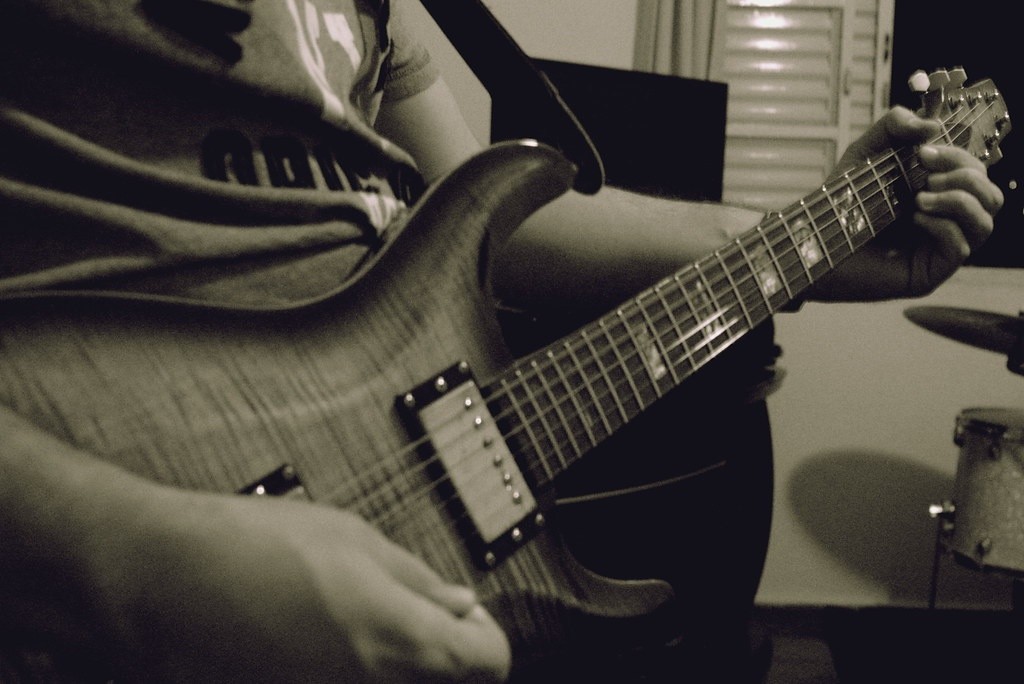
[900, 303, 1023, 353]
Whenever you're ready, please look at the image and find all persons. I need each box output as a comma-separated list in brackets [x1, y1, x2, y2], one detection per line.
[2, 0, 1006, 684]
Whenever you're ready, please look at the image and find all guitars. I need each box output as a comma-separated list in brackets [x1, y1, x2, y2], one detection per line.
[2, 59, 1009, 682]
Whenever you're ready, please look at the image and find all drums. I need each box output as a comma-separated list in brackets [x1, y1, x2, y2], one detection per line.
[944, 403, 1023, 571]
